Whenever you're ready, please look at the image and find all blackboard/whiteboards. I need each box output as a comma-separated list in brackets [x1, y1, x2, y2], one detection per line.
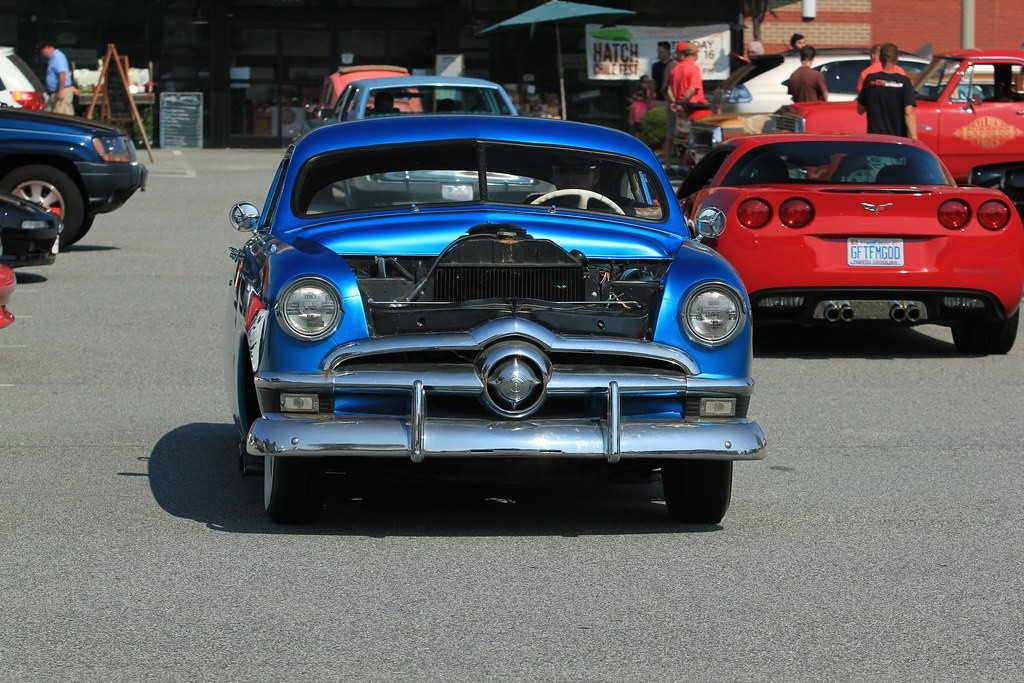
[159, 91, 203, 149]
[102, 55, 135, 122]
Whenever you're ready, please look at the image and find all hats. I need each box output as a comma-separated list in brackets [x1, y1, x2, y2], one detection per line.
[676, 42, 687, 52]
[685, 43, 700, 54]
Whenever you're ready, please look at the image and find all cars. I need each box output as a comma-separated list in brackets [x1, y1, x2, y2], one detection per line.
[229, 66, 768, 524]
[0, 47, 147, 328]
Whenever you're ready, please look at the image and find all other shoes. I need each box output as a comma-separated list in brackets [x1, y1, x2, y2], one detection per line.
[665, 168, 676, 176]
[679, 169, 687, 175]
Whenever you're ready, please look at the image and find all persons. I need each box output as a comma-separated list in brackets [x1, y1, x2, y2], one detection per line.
[790, 33, 805, 48]
[523, 155, 636, 215]
[857, 43, 917, 139]
[40, 41, 79, 116]
[629, 41, 707, 173]
[788, 45, 827, 103]
[856, 44, 906, 91]
[748, 42, 764, 56]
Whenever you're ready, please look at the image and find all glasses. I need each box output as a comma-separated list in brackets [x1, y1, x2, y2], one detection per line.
[552, 162, 599, 177]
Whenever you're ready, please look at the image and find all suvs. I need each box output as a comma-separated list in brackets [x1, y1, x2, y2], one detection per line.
[690, 43, 1024, 213]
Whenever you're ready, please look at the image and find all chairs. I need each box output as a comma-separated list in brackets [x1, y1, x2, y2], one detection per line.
[743, 155, 789, 181]
[875, 164, 919, 184]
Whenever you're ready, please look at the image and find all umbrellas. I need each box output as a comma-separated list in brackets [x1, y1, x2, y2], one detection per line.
[477, 0, 636, 120]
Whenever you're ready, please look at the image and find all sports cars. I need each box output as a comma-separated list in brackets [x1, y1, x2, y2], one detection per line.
[674, 133, 1024, 354]
